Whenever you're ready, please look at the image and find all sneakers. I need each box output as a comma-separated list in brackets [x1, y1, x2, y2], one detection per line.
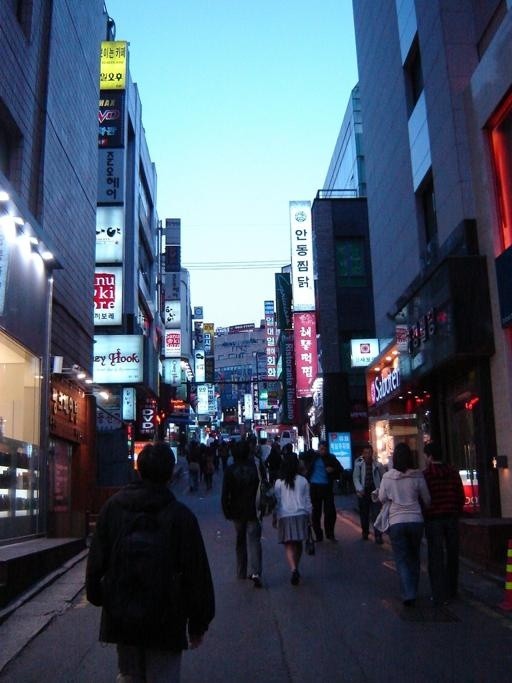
[376, 535, 382, 544]
[252, 573, 263, 585]
[290, 569, 299, 585]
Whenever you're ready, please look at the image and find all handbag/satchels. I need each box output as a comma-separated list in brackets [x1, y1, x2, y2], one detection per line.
[255, 482, 269, 519]
[306, 531, 315, 555]
[189, 462, 200, 471]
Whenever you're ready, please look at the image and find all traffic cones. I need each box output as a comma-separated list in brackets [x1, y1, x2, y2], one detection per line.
[495, 538, 512, 610]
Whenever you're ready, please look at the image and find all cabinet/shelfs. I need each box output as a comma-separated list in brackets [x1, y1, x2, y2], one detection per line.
[0, 433, 40, 539]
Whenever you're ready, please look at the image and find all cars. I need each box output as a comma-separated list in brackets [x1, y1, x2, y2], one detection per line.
[221, 432, 231, 442]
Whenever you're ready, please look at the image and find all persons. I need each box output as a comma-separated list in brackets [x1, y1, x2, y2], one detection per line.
[85, 443, 215, 683]
[221, 436, 345, 586]
[354, 446, 387, 544]
[183, 438, 229, 493]
[378, 441, 466, 612]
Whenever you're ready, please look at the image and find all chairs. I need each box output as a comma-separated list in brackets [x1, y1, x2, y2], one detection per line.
[84, 510, 100, 548]
